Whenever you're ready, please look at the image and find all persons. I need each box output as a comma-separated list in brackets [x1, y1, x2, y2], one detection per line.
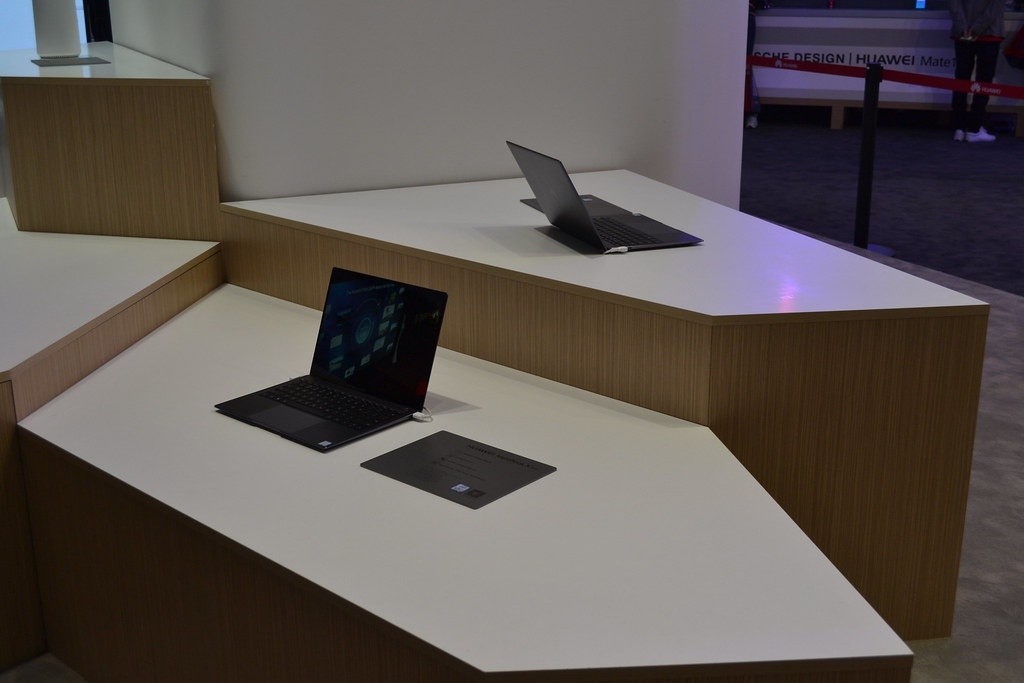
[950, 0, 1005, 142]
[737, 0, 768, 128]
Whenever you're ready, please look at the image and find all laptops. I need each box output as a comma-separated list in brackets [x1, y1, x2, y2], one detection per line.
[506, 140, 704, 254]
[212, 266, 447, 452]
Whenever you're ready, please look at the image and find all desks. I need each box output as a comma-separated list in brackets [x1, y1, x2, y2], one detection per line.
[17, 279, 917, 683]
[0, 41, 222, 243]
[215, 165, 992, 646]
[0, 198, 222, 426]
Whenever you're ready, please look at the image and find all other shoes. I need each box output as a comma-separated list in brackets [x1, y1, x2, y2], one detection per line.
[745, 115, 758, 128]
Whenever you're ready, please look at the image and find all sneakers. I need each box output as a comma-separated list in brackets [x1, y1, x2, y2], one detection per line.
[953, 129, 965, 142]
[965, 126, 996, 142]
